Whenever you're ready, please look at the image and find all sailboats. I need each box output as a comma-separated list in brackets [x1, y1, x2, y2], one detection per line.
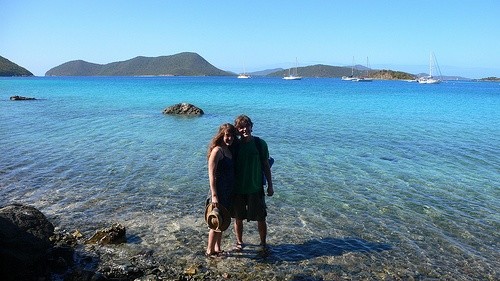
[418, 46, 444, 84]
[281, 56, 302, 80]
[342, 54, 373, 82]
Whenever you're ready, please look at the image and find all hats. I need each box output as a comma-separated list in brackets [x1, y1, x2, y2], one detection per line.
[205, 202, 231, 233]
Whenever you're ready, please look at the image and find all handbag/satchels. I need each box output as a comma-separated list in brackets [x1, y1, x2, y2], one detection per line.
[263, 157, 274, 186]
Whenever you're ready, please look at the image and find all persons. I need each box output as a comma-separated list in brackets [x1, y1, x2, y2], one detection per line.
[204, 114, 274, 256]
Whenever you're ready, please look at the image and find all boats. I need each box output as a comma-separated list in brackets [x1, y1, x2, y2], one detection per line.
[237, 64, 251, 79]
